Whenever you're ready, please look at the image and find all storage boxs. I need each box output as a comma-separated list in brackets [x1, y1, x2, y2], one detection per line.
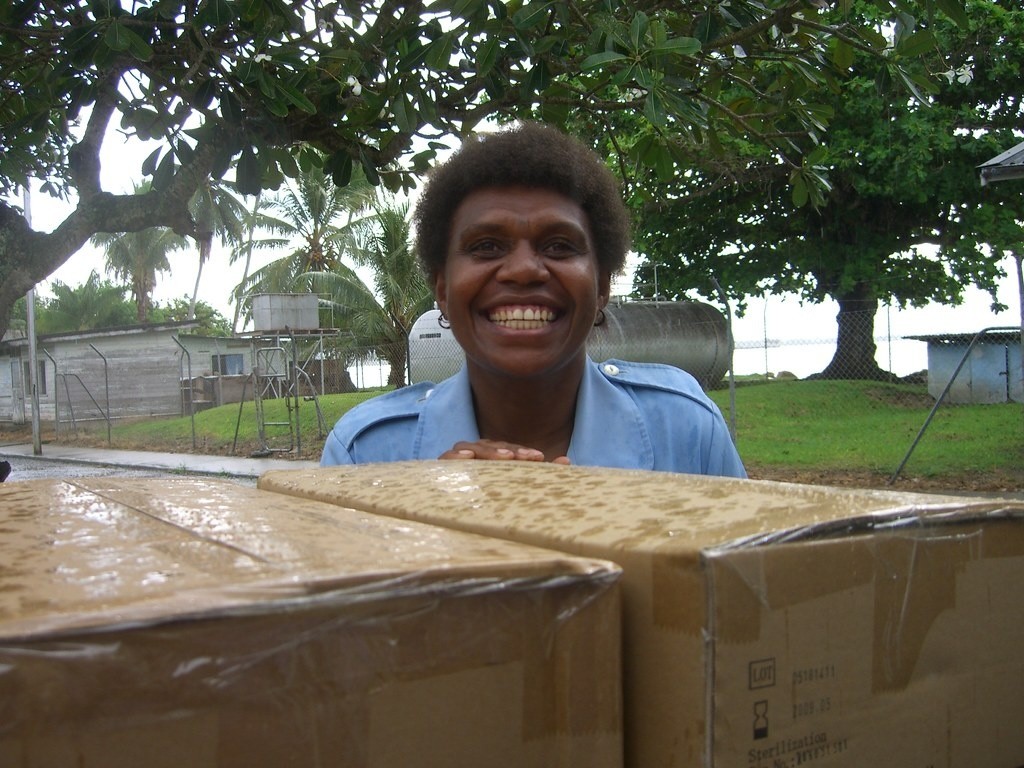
[0, 475, 627, 766]
[256, 457, 1016, 766]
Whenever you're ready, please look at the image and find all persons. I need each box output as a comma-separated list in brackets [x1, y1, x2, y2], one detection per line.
[323, 123, 751, 479]
